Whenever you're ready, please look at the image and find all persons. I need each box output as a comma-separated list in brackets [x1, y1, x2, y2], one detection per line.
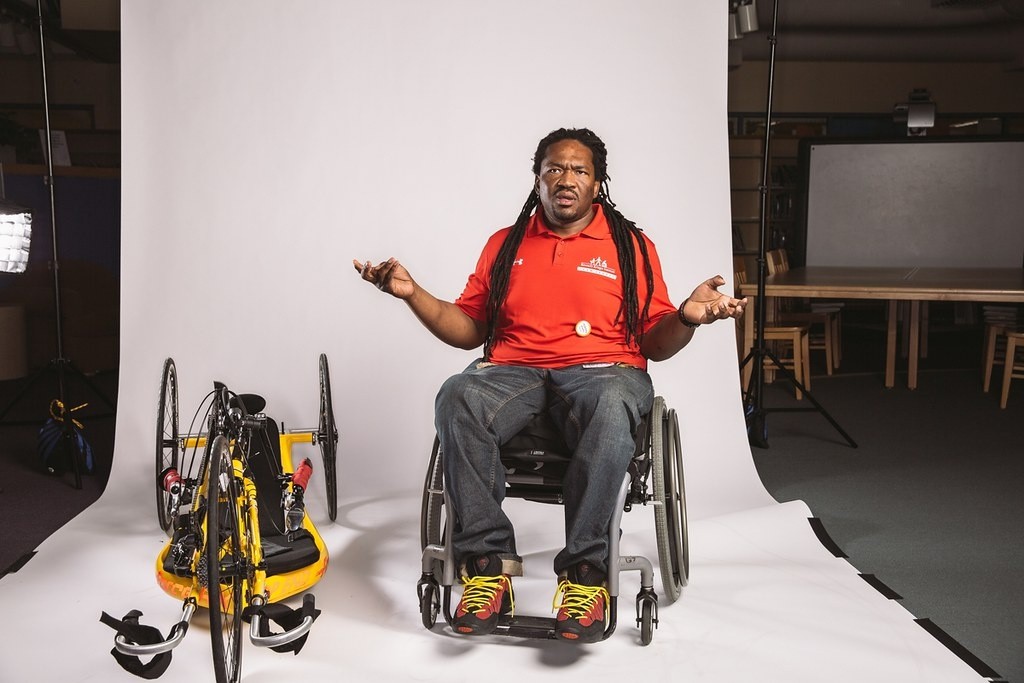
[349, 126, 749, 643]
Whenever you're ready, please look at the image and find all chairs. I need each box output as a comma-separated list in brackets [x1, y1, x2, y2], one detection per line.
[734, 248, 845, 400]
[980, 304, 1024, 409]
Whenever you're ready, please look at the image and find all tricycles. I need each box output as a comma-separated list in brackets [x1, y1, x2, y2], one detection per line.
[100, 352, 341, 683]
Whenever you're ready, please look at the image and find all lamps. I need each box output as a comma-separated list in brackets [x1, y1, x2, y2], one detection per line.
[728, 0, 759, 40]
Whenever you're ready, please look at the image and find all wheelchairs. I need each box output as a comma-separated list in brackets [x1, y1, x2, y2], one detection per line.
[415, 390, 691, 646]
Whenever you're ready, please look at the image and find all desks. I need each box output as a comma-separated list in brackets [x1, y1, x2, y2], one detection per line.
[739, 266, 1024, 400]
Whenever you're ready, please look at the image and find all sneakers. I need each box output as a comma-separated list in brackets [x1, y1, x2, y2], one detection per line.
[551, 561, 610, 642]
[450, 554, 514, 637]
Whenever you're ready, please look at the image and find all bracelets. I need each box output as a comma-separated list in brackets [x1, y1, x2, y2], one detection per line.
[678, 297, 701, 329]
